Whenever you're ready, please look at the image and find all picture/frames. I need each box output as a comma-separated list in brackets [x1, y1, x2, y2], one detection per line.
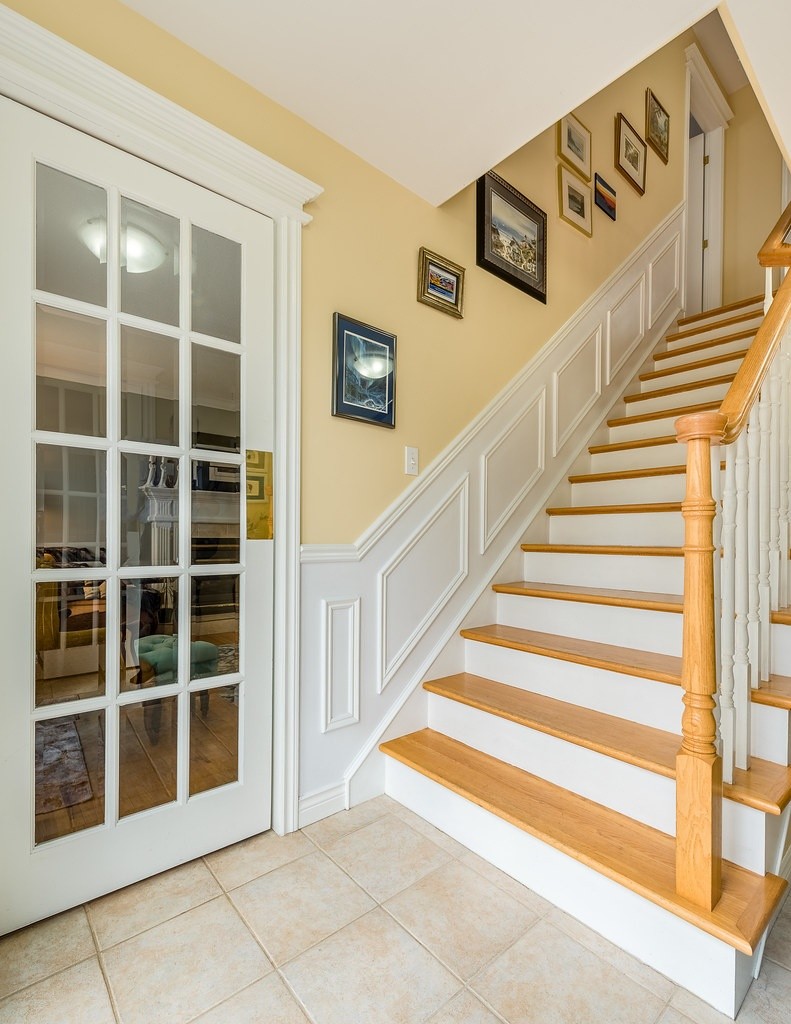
[556, 112, 593, 183]
[614, 112, 648, 196]
[330, 310, 398, 429]
[645, 86, 670, 166]
[476, 170, 548, 305]
[417, 246, 467, 320]
[558, 163, 594, 239]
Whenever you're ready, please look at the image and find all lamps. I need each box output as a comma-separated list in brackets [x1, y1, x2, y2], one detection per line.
[77, 210, 168, 276]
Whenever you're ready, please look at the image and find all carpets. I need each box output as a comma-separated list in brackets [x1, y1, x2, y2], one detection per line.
[35, 721, 94, 815]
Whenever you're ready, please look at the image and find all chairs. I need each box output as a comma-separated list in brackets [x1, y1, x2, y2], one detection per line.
[96, 587, 218, 746]
